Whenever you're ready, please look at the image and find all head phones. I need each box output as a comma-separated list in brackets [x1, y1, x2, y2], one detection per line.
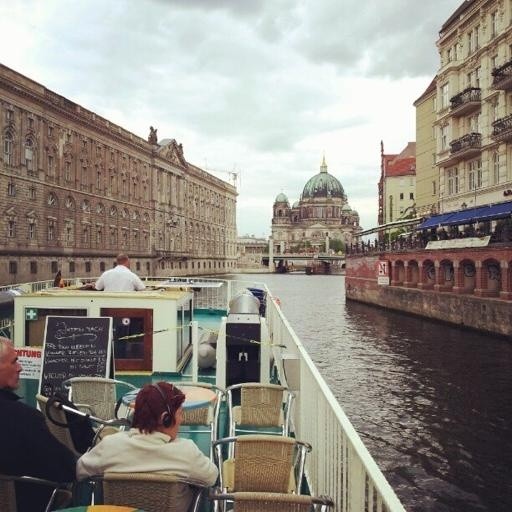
[152, 382, 176, 429]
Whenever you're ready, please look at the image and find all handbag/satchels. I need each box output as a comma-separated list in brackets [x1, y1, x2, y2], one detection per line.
[44, 392, 102, 454]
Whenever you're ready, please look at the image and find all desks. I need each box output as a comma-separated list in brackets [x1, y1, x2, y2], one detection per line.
[123, 387, 216, 432]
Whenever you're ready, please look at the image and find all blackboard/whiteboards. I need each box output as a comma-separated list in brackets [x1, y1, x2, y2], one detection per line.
[36, 315, 114, 413]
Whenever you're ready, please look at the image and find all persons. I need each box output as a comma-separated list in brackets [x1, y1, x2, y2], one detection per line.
[92, 252, 146, 291]
[414, 227, 497, 246]
[0, 335, 76, 512]
[76, 381, 219, 488]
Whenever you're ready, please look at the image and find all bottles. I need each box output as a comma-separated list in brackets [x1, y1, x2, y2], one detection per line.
[59, 279, 64, 288]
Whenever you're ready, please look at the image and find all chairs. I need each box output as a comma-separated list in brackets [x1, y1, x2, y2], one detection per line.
[212, 435, 312, 510]
[224, 382, 296, 459]
[37, 394, 130, 512]
[207, 486, 335, 512]
[0, 473, 96, 512]
[170, 382, 226, 442]
[89, 474, 206, 512]
[64, 378, 136, 420]
